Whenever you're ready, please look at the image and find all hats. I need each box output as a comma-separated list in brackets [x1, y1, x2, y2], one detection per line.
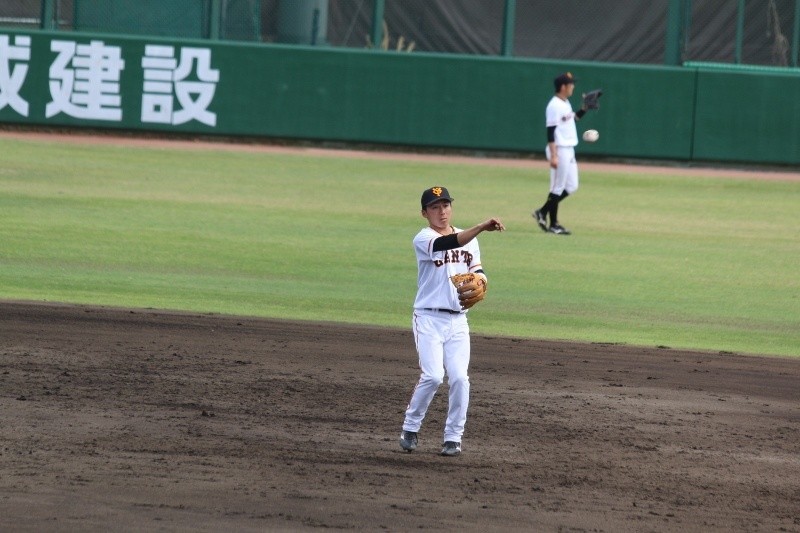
[422, 187, 455, 207]
[553, 72, 578, 87]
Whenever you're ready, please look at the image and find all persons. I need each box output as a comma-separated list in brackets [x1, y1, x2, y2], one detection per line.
[530, 71, 603, 235]
[399, 186, 507, 457]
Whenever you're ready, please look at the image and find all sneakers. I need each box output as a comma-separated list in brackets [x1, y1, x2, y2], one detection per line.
[531, 209, 548, 232]
[442, 441, 461, 454]
[549, 223, 570, 235]
[400, 430, 418, 450]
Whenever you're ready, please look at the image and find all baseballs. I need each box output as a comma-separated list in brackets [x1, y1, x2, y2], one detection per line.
[583, 129, 599, 142]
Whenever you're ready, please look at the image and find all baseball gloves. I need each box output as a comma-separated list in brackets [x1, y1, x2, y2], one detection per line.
[450, 273, 488, 310]
[582, 90, 603, 109]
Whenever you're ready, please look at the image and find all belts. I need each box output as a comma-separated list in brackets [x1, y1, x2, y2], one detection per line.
[425, 308, 460, 314]
